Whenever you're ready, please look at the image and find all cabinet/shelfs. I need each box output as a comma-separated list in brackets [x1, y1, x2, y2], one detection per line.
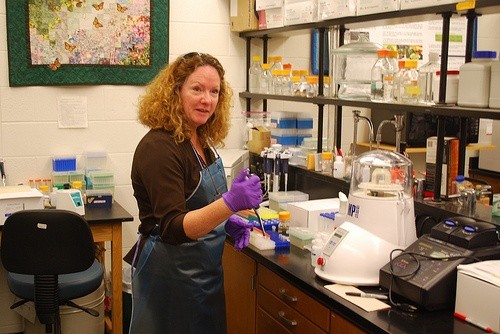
[222, 242, 492, 334]
[239, 0, 500, 229]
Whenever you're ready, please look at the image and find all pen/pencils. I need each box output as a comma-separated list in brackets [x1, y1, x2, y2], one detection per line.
[345, 292, 388, 299]
[454, 312, 491, 333]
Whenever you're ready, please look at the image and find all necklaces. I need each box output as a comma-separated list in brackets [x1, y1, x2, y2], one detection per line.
[200, 154, 228, 200]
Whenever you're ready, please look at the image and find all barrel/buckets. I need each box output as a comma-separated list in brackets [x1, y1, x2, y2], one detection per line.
[23, 279, 105, 334]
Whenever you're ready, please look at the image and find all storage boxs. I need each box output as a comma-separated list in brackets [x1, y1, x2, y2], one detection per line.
[424, 136, 459, 198]
[289, 198, 340, 249]
[268, 191, 309, 212]
[454, 261, 500, 334]
[52, 155, 114, 206]
[229, 0, 464, 32]
[247, 111, 313, 154]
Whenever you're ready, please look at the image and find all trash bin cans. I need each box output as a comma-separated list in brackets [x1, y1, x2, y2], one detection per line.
[121, 267, 136, 334]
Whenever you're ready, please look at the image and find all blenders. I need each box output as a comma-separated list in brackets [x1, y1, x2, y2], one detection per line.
[314, 148, 419, 288]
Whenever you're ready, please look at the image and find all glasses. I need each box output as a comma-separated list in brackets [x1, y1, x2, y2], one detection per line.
[173, 52, 202, 73]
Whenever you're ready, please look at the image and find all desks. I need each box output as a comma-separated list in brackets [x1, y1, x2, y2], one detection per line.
[84, 201, 134, 334]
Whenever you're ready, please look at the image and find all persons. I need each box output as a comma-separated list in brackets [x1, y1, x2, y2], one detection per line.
[123, 52, 264, 334]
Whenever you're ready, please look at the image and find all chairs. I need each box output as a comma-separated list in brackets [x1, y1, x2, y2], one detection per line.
[0, 210, 103, 334]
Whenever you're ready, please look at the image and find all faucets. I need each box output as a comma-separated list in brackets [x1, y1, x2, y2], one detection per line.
[353, 110, 374, 156]
[376, 114, 407, 155]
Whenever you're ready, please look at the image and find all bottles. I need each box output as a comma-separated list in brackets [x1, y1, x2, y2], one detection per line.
[277, 211, 290, 240]
[332, 34, 500, 109]
[322, 153, 345, 179]
[248, 56, 329, 97]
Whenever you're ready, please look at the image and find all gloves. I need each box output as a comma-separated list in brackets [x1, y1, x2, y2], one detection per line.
[223, 215, 253, 251]
[221, 167, 264, 213]
